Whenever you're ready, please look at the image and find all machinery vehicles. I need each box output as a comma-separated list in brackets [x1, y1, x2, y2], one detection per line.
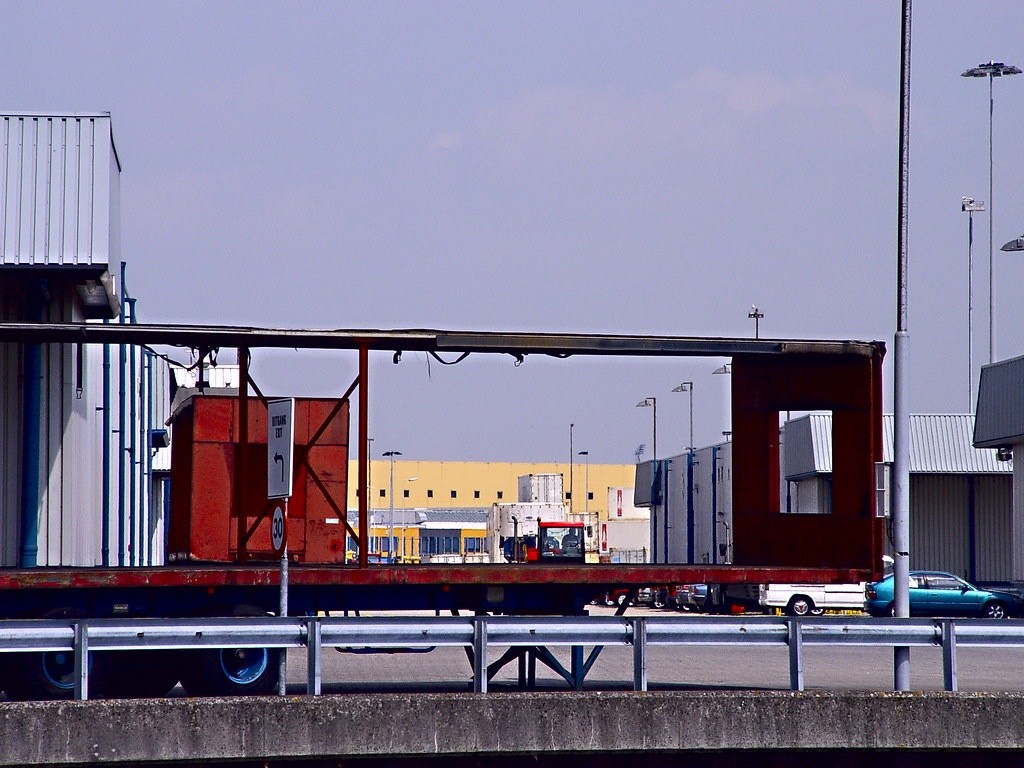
[507, 516, 586, 565]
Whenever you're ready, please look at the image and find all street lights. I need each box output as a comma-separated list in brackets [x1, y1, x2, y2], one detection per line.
[748, 305, 765, 340]
[958, 61, 1024, 363]
[959, 195, 987, 417]
[636, 397, 658, 564]
[367, 437, 375, 553]
[579, 451, 590, 513]
[381, 451, 402, 560]
[672, 380, 694, 563]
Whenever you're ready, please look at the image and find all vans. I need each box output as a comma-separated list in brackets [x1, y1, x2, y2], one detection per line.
[757, 554, 918, 616]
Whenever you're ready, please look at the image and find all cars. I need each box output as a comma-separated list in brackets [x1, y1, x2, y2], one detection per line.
[588, 560, 775, 615]
[864, 569, 1024, 619]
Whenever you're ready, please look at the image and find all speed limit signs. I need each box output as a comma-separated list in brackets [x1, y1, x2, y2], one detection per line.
[271, 505, 288, 553]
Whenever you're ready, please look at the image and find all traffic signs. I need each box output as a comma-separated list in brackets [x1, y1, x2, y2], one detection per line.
[266, 398, 295, 499]
